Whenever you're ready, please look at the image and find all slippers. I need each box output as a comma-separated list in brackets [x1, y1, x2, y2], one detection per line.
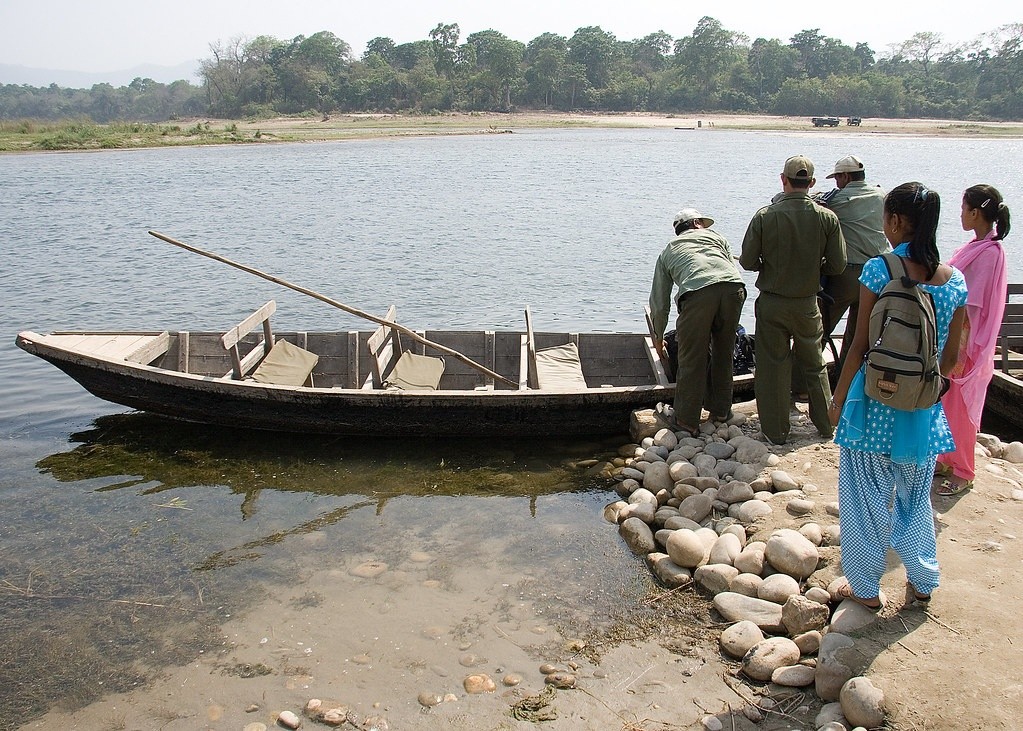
[908, 580, 932, 603]
[837, 584, 884, 613]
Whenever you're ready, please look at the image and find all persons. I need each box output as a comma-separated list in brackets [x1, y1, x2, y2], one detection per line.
[648, 207, 747, 432]
[933, 183, 1011, 495]
[827, 182, 969, 613]
[739, 155, 846, 445]
[771, 155, 891, 403]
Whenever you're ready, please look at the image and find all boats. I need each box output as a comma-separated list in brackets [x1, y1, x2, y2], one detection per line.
[981, 281, 1023, 433]
[15, 327, 848, 440]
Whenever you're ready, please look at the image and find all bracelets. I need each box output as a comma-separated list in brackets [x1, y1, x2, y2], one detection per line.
[829, 396, 842, 410]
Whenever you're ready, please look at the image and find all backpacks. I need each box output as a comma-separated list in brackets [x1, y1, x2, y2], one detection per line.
[863, 252, 950, 412]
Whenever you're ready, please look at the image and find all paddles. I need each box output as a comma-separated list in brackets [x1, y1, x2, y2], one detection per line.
[147, 229, 518, 388]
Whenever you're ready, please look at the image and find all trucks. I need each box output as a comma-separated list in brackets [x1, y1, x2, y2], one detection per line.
[811, 117, 840, 128]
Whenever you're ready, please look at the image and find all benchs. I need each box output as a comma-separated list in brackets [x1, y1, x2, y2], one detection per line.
[220, 299, 320, 386]
[518, 306, 588, 392]
[994, 284, 1023, 376]
[361, 305, 446, 391]
[642, 305, 674, 387]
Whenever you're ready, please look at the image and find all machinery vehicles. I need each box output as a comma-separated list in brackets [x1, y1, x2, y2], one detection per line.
[847, 116, 862, 127]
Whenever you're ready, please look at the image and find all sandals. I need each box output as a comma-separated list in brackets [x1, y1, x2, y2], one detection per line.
[712, 410, 733, 422]
[934, 459, 954, 477]
[936, 474, 974, 496]
[655, 401, 701, 438]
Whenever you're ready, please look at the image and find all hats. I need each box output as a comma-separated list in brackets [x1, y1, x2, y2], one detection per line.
[826, 154, 865, 179]
[673, 208, 714, 230]
[784, 155, 814, 180]
